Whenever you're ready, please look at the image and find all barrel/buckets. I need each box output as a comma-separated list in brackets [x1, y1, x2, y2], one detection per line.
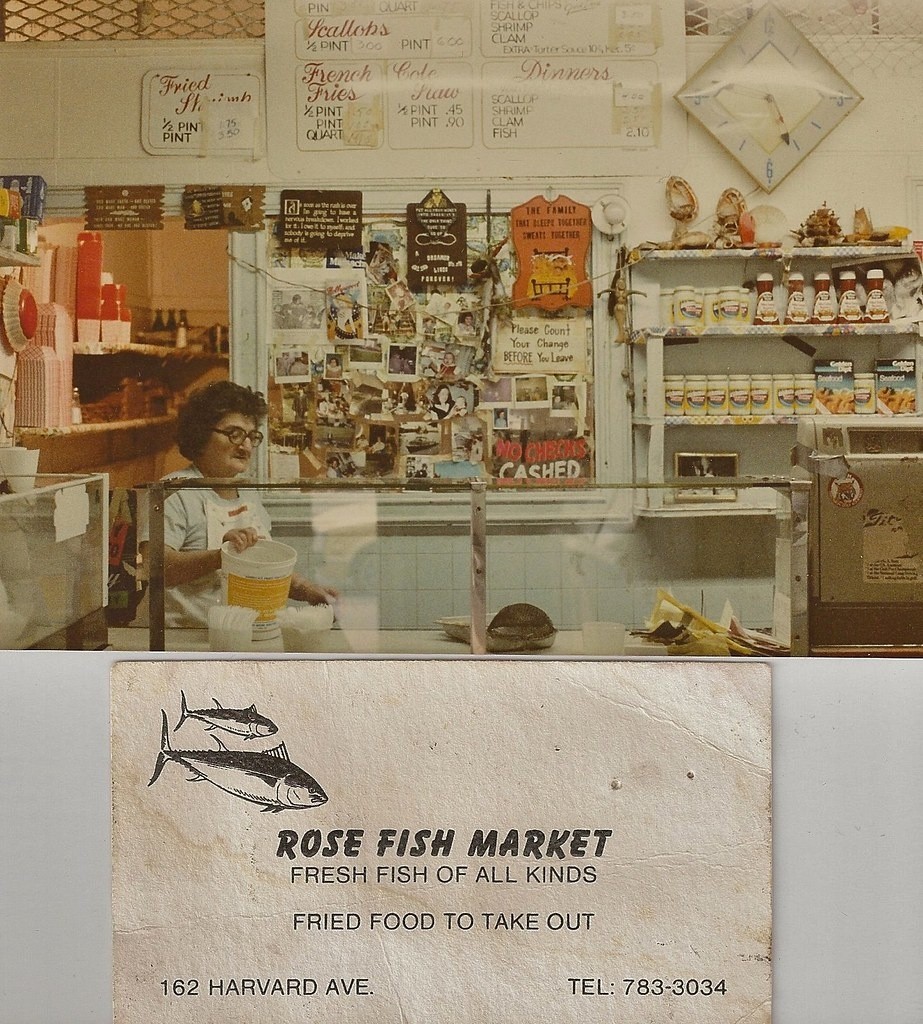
[219, 540, 297, 641]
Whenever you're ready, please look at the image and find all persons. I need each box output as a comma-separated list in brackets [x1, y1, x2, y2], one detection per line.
[278, 256, 475, 478]
[596, 278, 648, 342]
[131, 380, 341, 628]
[690, 460, 714, 477]
[553, 394, 577, 410]
[495, 409, 506, 427]
[826, 433, 840, 448]
[519, 386, 547, 401]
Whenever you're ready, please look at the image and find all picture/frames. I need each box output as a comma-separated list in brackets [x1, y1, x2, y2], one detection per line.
[671, 451, 738, 504]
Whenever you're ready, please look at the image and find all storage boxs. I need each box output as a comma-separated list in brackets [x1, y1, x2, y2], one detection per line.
[874, 358, 916, 415]
[0, 175, 48, 256]
[806, 453, 923, 645]
[813, 359, 854, 415]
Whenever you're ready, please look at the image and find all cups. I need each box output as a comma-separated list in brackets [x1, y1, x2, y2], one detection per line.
[582, 621, 625, 656]
[280, 626, 332, 652]
[208, 627, 252, 653]
[0, 449, 40, 494]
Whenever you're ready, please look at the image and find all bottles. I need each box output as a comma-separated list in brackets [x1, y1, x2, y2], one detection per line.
[752, 272, 779, 324]
[663, 374, 816, 414]
[837, 271, 864, 322]
[864, 270, 889, 322]
[166, 308, 176, 331]
[810, 271, 837, 323]
[152, 308, 164, 332]
[179, 308, 188, 330]
[660, 285, 750, 327]
[784, 271, 811, 324]
[175, 322, 187, 348]
[853, 373, 876, 414]
[71, 387, 83, 426]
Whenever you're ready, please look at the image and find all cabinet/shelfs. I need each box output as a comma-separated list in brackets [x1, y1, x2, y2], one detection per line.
[15, 341, 227, 439]
[626, 244, 922, 517]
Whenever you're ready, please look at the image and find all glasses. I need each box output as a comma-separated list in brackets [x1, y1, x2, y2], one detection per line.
[212, 426, 264, 449]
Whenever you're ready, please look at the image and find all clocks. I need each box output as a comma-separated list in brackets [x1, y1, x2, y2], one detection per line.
[674, 1, 865, 195]
